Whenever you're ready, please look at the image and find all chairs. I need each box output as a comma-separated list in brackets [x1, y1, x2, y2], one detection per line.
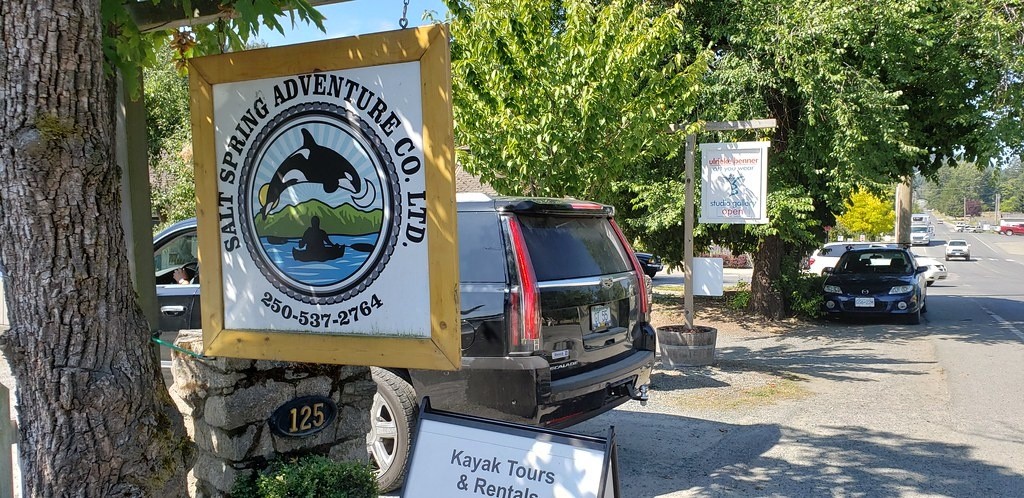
[889, 258, 904, 272]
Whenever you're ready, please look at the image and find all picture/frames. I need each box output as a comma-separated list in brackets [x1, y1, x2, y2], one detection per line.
[185, 24, 463, 369]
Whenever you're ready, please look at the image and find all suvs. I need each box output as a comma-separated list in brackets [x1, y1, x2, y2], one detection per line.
[822, 244, 927, 325]
[153, 192, 656, 493]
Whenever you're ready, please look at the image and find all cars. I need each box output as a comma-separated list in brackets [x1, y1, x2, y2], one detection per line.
[944, 240, 971, 261]
[635, 253, 662, 278]
[954, 222, 983, 234]
[909, 212, 933, 246]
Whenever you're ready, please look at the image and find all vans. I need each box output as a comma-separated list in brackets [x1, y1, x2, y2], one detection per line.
[799, 242, 948, 285]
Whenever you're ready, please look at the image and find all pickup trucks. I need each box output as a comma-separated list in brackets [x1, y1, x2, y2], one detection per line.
[1000, 224, 1024, 236]
